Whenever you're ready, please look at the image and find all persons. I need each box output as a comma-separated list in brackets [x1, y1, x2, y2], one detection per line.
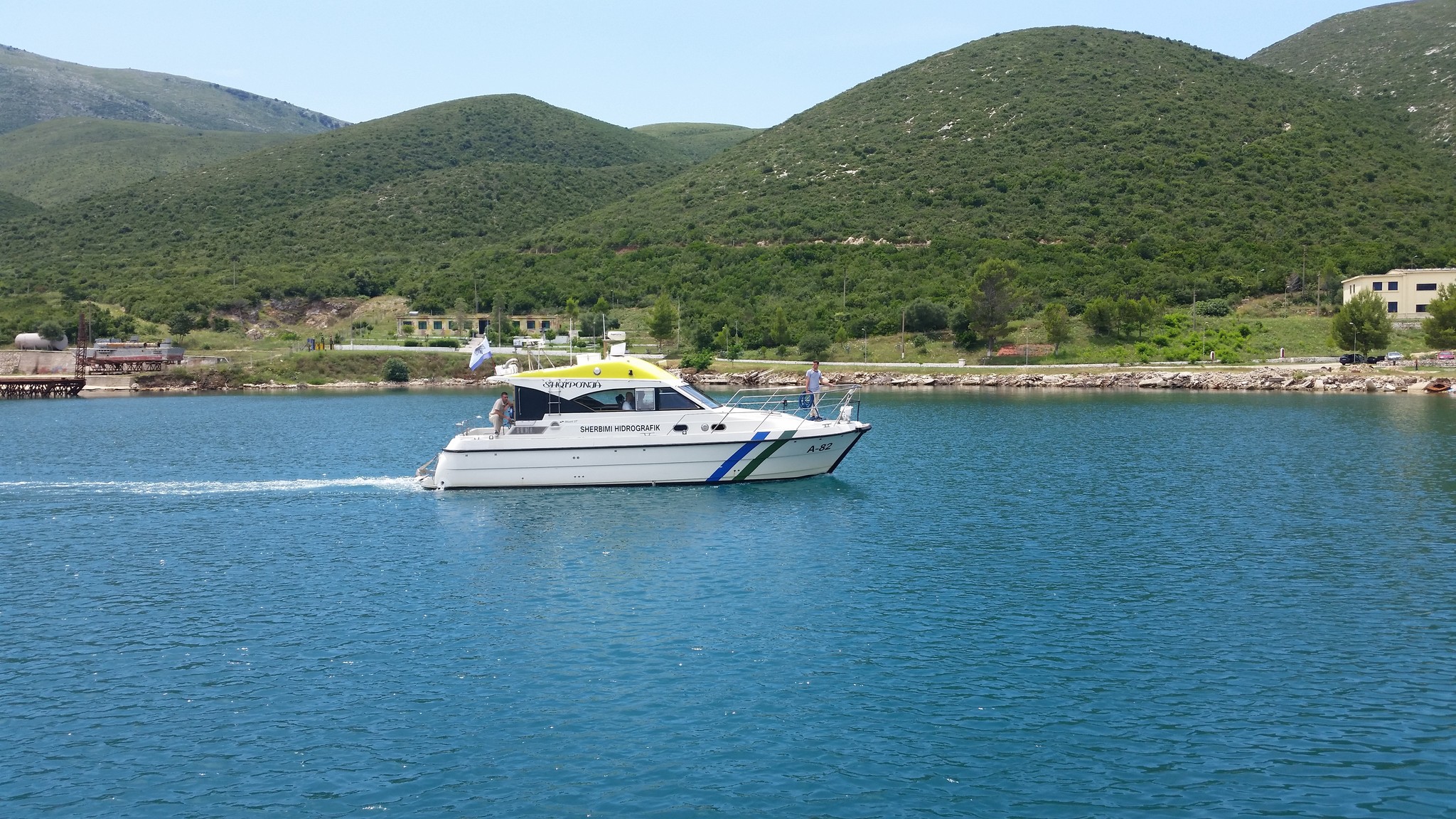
[638, 391, 650, 409]
[489, 392, 514, 437]
[622, 392, 634, 410]
[806, 359, 834, 418]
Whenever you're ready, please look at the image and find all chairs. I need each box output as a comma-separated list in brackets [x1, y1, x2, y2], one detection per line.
[616, 394, 625, 410]
[630, 397, 635, 410]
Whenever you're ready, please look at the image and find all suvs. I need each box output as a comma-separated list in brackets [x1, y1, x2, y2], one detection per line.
[1339, 353, 1369, 365]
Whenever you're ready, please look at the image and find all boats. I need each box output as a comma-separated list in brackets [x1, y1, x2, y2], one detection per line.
[1426, 382, 1453, 392]
[415, 310, 874, 492]
[68, 335, 185, 364]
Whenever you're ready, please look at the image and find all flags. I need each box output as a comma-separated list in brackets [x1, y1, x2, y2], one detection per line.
[469, 337, 492, 371]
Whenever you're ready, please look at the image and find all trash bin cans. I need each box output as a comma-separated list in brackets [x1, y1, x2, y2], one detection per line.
[959, 359, 966, 365]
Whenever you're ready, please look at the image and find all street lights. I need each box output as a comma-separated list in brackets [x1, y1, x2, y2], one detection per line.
[593, 322, 596, 353]
[1257, 269, 1265, 283]
[1350, 322, 1356, 365]
[735, 321, 738, 345]
[1025, 328, 1032, 364]
[611, 290, 614, 309]
[862, 328, 866, 363]
[1202, 322, 1209, 360]
[1284, 286, 1290, 318]
[1411, 256, 1418, 269]
[722, 327, 728, 359]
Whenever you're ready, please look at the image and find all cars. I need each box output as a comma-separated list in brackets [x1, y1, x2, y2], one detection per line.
[1437, 351, 1455, 360]
[1385, 352, 1402, 360]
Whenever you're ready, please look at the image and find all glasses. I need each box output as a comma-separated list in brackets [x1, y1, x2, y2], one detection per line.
[504, 397, 508, 398]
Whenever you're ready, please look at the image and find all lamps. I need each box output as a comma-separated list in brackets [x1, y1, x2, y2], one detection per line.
[628, 370, 633, 375]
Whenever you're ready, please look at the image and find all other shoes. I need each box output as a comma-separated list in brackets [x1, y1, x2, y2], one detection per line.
[494, 430, 499, 436]
[815, 418, 820, 420]
[810, 416, 815, 418]
